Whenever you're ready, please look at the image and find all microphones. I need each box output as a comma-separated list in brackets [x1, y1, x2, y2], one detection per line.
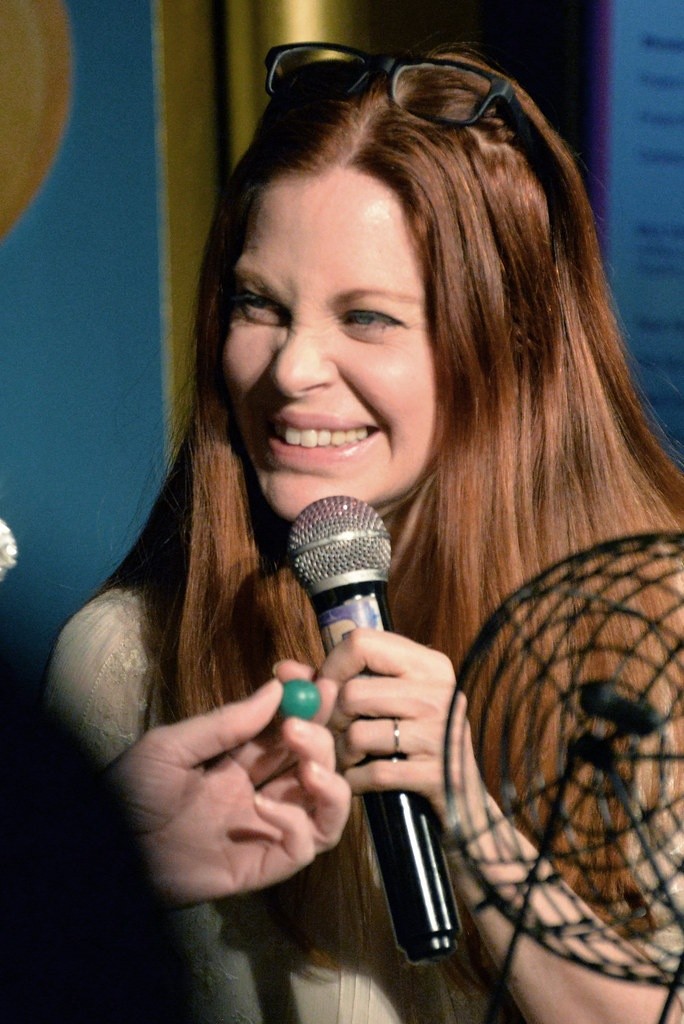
[289, 496, 463, 969]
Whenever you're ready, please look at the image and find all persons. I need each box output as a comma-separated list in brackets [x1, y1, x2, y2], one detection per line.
[35, 48, 684, 1024]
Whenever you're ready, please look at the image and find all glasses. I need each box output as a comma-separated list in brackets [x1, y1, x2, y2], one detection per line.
[264, 41, 563, 199]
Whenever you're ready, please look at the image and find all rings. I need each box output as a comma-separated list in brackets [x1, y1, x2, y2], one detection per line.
[389, 718, 402, 756]
